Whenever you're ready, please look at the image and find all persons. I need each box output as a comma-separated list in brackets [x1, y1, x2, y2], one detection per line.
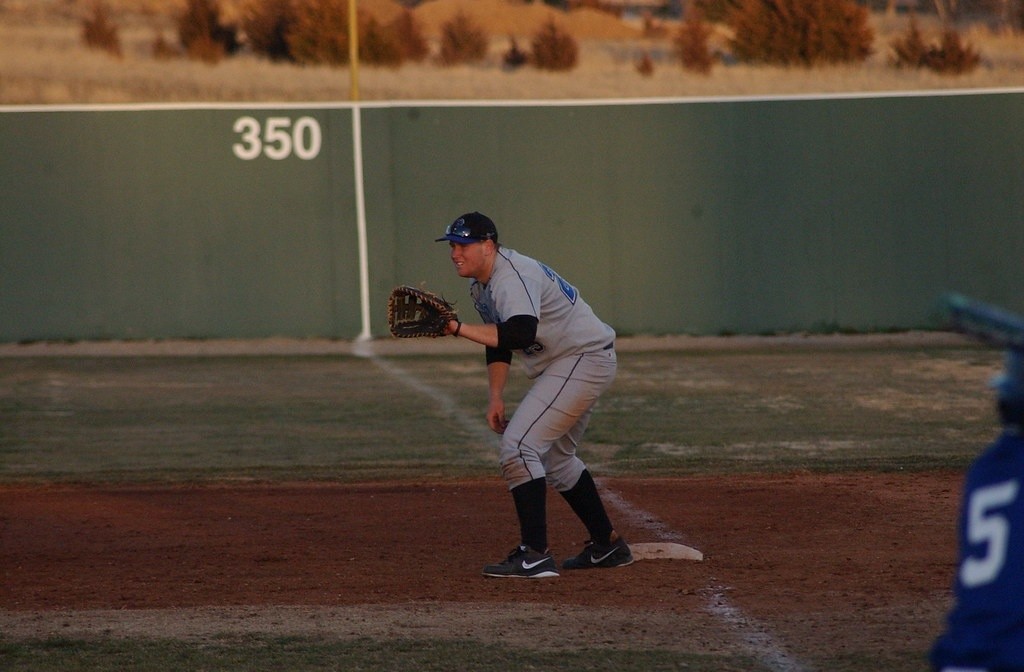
[923, 342, 1023, 672]
[385, 210, 633, 579]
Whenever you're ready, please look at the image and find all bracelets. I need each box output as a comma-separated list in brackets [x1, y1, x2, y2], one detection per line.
[452, 322, 461, 337]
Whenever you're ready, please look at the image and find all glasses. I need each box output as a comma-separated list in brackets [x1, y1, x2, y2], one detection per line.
[444, 223, 496, 238]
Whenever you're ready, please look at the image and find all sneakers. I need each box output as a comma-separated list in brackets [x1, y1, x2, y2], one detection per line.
[563, 535, 635, 569]
[482, 545, 560, 578]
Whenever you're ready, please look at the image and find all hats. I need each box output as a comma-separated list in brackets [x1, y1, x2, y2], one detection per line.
[435, 212, 498, 246]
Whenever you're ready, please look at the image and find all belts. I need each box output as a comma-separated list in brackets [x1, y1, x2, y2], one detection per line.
[603, 341, 614, 350]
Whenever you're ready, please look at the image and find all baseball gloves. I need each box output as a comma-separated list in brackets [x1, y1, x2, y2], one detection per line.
[386, 280, 460, 341]
